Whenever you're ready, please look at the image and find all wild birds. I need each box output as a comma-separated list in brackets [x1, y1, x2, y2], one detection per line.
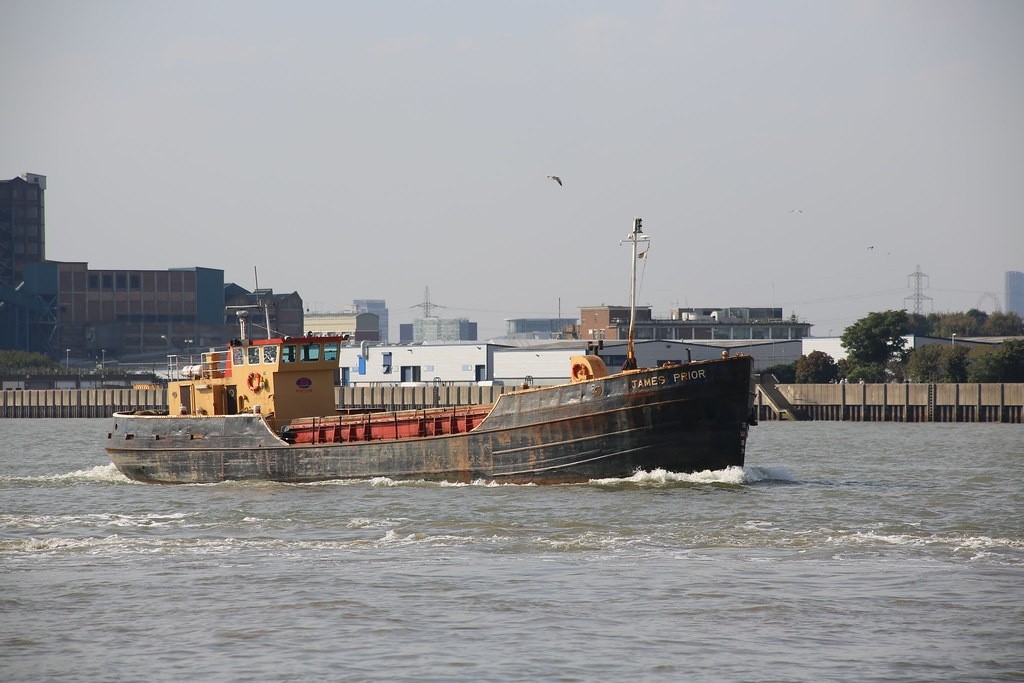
[866, 245, 875, 249]
[789, 209, 803, 215]
[546, 175, 563, 188]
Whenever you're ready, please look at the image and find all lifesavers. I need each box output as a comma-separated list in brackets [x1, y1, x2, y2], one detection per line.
[247, 372, 261, 393]
[572, 362, 588, 382]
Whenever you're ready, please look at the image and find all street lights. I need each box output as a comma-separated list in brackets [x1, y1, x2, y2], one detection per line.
[951, 332, 958, 352]
[184, 340, 194, 365]
[161, 335, 174, 380]
[66, 348, 71, 370]
[102, 348, 108, 372]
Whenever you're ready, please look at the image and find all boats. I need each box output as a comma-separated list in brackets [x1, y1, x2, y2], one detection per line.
[102, 216, 763, 485]
